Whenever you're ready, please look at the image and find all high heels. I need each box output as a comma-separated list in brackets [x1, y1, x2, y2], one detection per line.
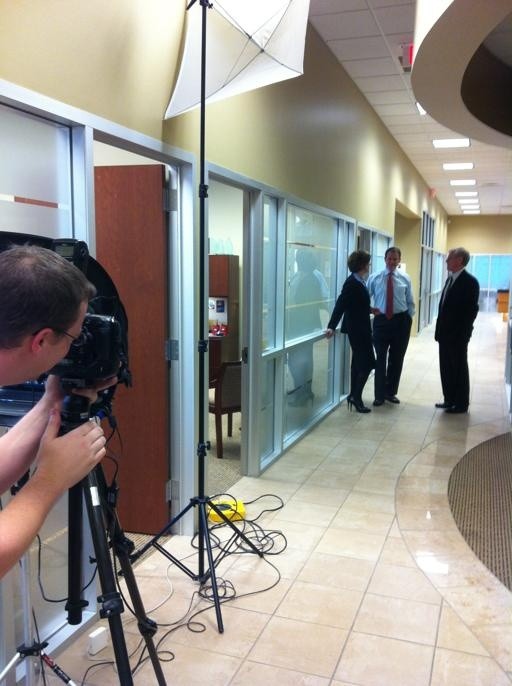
[347, 395, 371, 413]
[288, 392, 314, 408]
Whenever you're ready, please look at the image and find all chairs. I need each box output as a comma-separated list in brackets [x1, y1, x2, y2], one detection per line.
[208, 361, 241, 458]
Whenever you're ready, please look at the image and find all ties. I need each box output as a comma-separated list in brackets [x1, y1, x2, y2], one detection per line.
[449, 277, 453, 287]
[386, 273, 393, 320]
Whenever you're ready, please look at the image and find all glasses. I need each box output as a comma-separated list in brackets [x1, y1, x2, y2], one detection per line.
[32, 325, 88, 347]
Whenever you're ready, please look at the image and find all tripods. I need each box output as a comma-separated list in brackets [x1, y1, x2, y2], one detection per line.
[117, 1, 266, 636]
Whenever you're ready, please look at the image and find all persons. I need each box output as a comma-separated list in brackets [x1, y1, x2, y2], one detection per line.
[286, 250, 322, 408]
[288, 248, 329, 309]
[321, 248, 381, 414]
[1, 239, 119, 583]
[367, 247, 416, 405]
[433, 246, 481, 414]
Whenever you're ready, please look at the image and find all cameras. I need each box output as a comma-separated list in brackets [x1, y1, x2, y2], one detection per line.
[51, 309, 134, 398]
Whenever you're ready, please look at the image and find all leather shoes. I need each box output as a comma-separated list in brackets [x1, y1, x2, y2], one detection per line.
[445, 406, 468, 413]
[373, 400, 384, 405]
[385, 395, 399, 403]
[436, 402, 453, 408]
